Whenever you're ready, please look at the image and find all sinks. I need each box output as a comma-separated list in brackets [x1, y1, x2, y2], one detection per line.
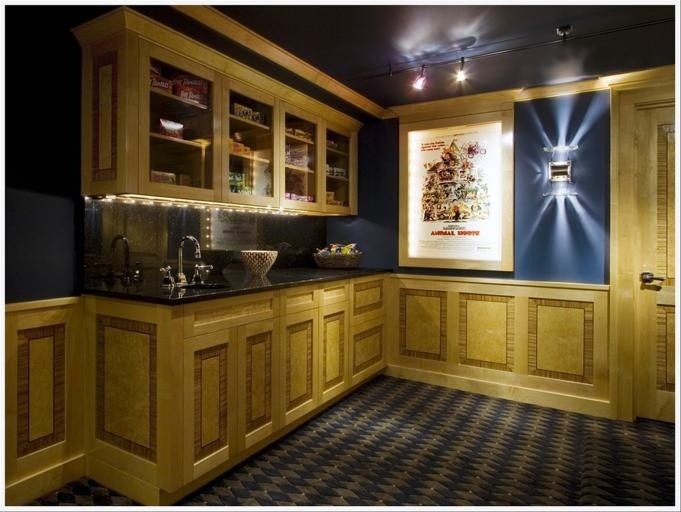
[182, 284, 232, 290]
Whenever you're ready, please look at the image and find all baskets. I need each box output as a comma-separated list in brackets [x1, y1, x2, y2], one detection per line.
[312, 249, 361, 269]
[272, 248, 306, 268]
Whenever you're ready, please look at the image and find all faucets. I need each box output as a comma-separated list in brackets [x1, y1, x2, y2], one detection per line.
[111, 234, 130, 276]
[178, 234, 201, 283]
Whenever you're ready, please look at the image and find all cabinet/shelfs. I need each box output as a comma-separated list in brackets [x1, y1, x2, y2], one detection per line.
[79, 267, 385, 504]
[71, 8, 364, 216]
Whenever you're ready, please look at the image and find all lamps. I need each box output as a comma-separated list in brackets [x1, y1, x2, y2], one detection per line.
[451, 56, 470, 85]
[411, 65, 428, 91]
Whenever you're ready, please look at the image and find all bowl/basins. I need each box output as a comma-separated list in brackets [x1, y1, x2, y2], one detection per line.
[313, 252, 363, 270]
[241, 250, 279, 280]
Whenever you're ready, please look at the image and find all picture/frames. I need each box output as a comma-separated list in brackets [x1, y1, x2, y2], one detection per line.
[397, 109, 514, 271]
